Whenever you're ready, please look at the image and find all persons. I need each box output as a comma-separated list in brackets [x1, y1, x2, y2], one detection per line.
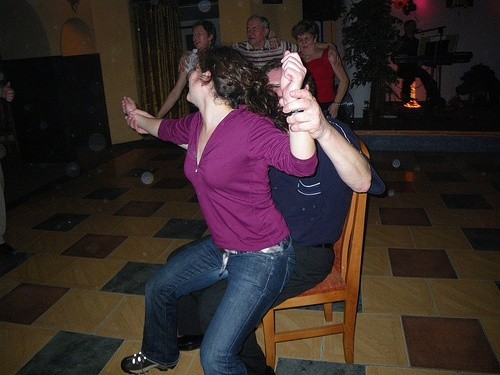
[155, 19, 217, 119]
[121, 44, 318, 375]
[291, 19, 350, 130]
[177, 15, 342, 72]
[390, 19, 446, 105]
[126, 59, 385, 375]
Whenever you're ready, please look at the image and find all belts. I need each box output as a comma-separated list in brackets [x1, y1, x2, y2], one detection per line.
[311, 242, 334, 249]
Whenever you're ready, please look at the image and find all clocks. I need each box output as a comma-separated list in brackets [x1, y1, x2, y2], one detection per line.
[198, 0, 212, 13]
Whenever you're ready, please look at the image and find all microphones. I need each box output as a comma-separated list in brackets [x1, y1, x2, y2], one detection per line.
[415, 28, 421, 32]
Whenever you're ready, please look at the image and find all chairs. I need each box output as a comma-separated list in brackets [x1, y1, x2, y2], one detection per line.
[262, 134, 369, 370]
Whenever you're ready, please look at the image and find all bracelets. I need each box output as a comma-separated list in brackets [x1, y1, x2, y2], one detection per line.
[333, 102, 340, 106]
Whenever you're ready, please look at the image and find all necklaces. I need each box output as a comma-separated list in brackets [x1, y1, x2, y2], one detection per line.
[299, 49, 314, 56]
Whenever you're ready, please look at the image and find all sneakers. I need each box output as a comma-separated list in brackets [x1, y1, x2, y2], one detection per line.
[121, 351, 177, 374]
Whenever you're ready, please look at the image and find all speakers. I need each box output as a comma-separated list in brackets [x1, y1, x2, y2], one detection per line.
[302, 0, 339, 21]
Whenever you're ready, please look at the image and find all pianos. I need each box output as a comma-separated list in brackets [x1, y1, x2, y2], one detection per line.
[391, 50, 474, 64]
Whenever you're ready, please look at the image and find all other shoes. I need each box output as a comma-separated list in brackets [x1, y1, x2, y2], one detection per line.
[178, 335, 203, 351]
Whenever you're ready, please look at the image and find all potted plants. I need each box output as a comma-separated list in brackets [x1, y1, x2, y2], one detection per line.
[342, 0, 403, 118]
